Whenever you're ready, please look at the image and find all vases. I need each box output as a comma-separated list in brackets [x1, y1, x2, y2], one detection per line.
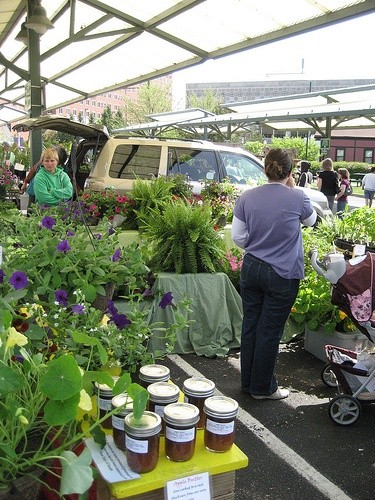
[0, 186, 8, 201]
[299, 324, 374, 370]
[0, 426, 64, 500]
[79, 213, 102, 226]
[106, 211, 127, 228]
[91, 282, 119, 310]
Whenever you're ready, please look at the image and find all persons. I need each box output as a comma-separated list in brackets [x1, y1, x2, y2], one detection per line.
[231, 148, 317, 398]
[336, 168, 353, 219]
[318, 158, 336, 212]
[296, 160, 313, 187]
[23, 146, 74, 207]
[361, 167, 375, 207]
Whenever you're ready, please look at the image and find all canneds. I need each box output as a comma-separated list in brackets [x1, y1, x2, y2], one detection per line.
[111, 393, 133, 450]
[146, 381, 180, 436]
[163, 402, 200, 462]
[182, 377, 215, 430]
[95, 376, 121, 429]
[203, 396, 239, 453]
[124, 411, 162, 474]
[138, 364, 171, 389]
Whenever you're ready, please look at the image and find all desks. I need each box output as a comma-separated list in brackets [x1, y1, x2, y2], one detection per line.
[75, 379, 248, 499]
[72, 223, 247, 269]
[117, 269, 247, 360]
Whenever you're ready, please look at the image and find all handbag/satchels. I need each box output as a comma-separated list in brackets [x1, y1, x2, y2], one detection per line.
[340, 180, 352, 196]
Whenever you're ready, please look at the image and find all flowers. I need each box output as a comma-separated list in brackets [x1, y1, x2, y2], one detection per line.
[0, 163, 368, 500]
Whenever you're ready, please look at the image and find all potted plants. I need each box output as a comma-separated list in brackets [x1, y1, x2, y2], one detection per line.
[334, 204, 375, 262]
[134, 205, 231, 273]
[132, 170, 189, 231]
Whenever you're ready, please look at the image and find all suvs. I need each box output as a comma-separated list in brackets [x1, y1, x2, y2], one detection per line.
[10, 113, 333, 233]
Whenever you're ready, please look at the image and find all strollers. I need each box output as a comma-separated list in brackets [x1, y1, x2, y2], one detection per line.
[305, 235, 375, 428]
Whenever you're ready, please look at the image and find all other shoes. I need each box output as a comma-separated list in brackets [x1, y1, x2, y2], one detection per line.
[251, 386, 289, 400]
